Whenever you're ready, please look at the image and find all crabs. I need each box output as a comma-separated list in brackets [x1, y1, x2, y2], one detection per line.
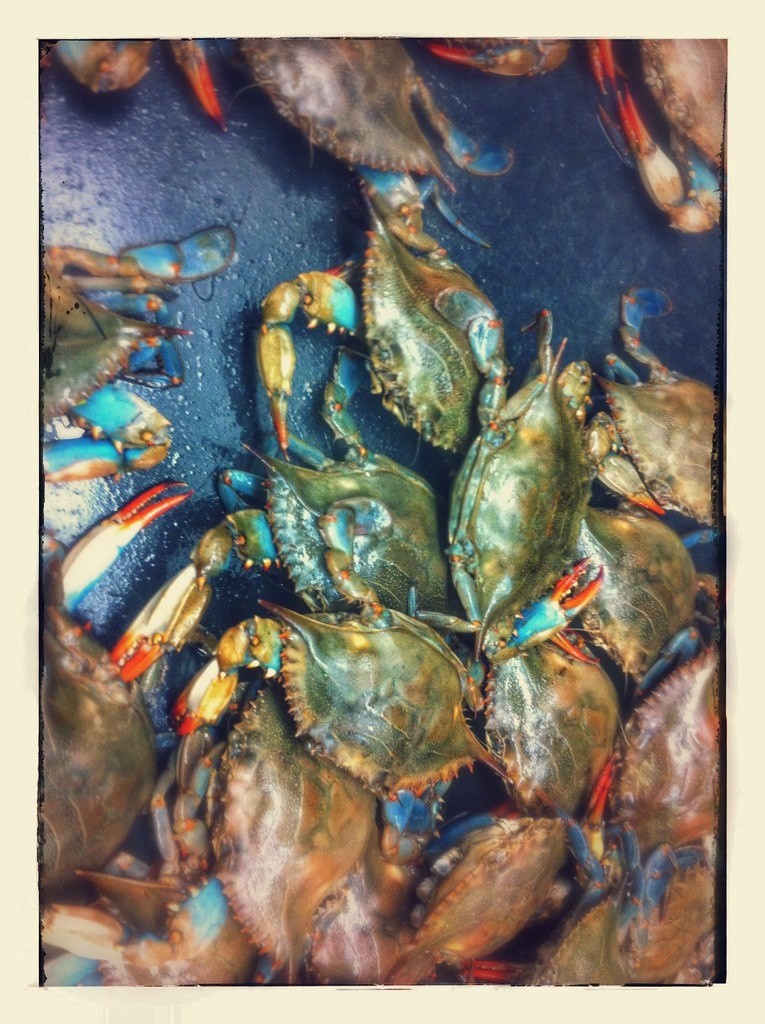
[38, 38, 722, 984]
